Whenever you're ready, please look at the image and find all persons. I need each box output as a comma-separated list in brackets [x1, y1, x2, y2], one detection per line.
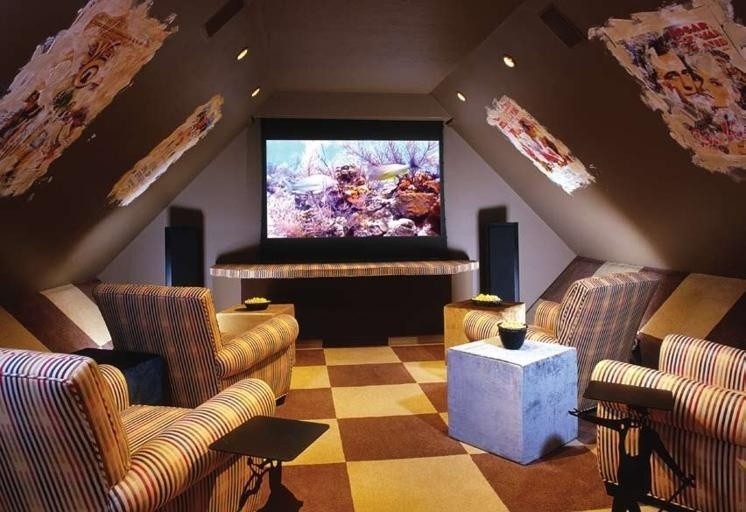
[635, 36, 699, 111]
[520, 118, 541, 152]
[683, 46, 745, 129]
[538, 136, 565, 159]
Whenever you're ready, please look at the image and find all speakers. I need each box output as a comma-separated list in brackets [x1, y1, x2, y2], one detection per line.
[478, 220, 520, 302]
[165, 207, 205, 288]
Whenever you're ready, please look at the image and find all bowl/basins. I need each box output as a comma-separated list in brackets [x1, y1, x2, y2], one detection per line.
[244, 302, 271, 310]
[497, 322, 527, 350]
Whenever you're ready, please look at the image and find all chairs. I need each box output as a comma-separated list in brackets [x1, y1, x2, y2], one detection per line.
[460, 265, 656, 418]
[91, 285, 301, 407]
[592, 329, 746, 510]
[0, 351, 278, 512]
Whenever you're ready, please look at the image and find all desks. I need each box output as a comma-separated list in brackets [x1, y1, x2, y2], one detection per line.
[443, 330, 582, 468]
[570, 379, 695, 509]
[444, 297, 527, 370]
[70, 349, 166, 410]
[205, 252, 479, 343]
[215, 302, 295, 335]
[204, 413, 331, 512]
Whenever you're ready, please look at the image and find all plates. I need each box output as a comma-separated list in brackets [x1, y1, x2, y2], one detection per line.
[472, 298, 504, 306]
